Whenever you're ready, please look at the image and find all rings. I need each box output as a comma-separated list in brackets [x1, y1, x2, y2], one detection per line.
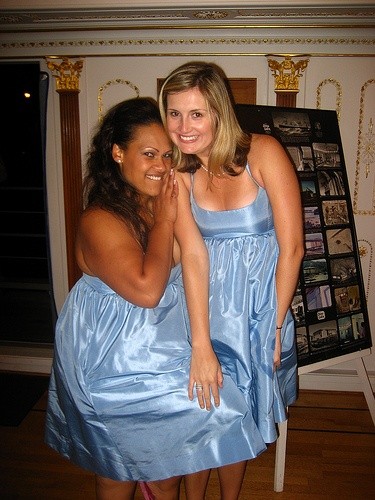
[196, 383, 203, 391]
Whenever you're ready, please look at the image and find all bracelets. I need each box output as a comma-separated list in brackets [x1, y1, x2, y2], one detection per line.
[276, 327, 282, 329]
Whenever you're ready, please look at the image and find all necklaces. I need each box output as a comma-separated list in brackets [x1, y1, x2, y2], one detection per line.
[199, 164, 226, 176]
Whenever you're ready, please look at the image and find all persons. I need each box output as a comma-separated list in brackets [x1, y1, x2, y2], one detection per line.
[46, 95, 195, 499]
[158, 60, 305, 500]
